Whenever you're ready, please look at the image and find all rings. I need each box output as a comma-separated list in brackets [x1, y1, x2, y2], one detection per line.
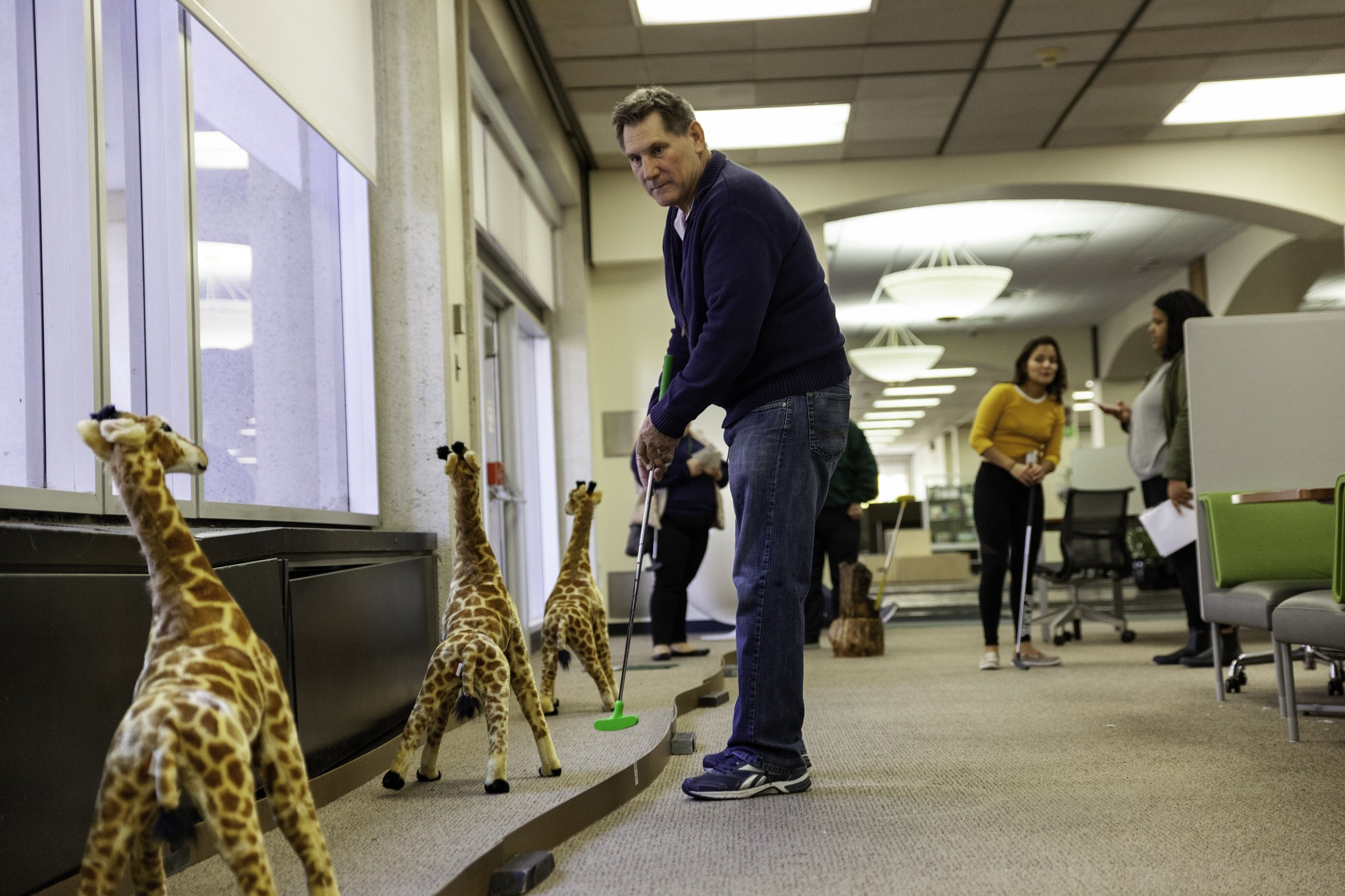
[656, 446, 668, 455]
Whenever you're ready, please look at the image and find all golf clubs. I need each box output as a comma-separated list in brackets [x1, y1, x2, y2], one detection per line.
[873, 496, 915, 623]
[1009, 449, 1043, 669]
[589, 465, 659, 728]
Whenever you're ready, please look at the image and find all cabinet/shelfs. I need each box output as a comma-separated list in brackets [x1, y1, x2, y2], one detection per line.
[0, 520, 439, 896]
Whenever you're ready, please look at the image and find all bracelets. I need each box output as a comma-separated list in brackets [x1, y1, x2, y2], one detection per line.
[1009, 460, 1017, 471]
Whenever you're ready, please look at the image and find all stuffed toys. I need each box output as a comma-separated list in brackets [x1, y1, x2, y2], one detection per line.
[381, 440, 563, 794]
[536, 478, 620, 714]
[70, 405, 340, 895]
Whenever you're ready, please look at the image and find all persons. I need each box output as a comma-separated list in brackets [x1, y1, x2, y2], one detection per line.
[631, 421, 730, 661]
[969, 338, 1068, 671]
[615, 86, 852, 800]
[801, 418, 879, 645]
[1097, 289, 1244, 667]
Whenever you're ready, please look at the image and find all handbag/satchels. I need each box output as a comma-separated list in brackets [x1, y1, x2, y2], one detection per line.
[1132, 556, 1178, 590]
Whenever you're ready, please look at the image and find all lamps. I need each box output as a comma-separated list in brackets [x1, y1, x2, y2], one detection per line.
[847, 230, 1014, 387]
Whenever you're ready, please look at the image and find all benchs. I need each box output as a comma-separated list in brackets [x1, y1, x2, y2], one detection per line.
[1199, 476, 1345, 743]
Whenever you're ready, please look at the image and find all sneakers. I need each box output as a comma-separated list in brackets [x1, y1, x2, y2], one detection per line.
[681, 753, 812, 800]
[703, 747, 812, 773]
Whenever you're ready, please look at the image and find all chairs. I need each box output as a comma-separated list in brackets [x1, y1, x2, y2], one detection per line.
[1034, 487, 1138, 644]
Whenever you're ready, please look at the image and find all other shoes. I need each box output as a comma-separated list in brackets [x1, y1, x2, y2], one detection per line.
[1152, 630, 1211, 665]
[1179, 627, 1244, 667]
[1015, 650, 1062, 666]
[651, 644, 710, 660]
[879, 603, 900, 624]
[979, 652, 1001, 668]
[803, 638, 822, 651]
